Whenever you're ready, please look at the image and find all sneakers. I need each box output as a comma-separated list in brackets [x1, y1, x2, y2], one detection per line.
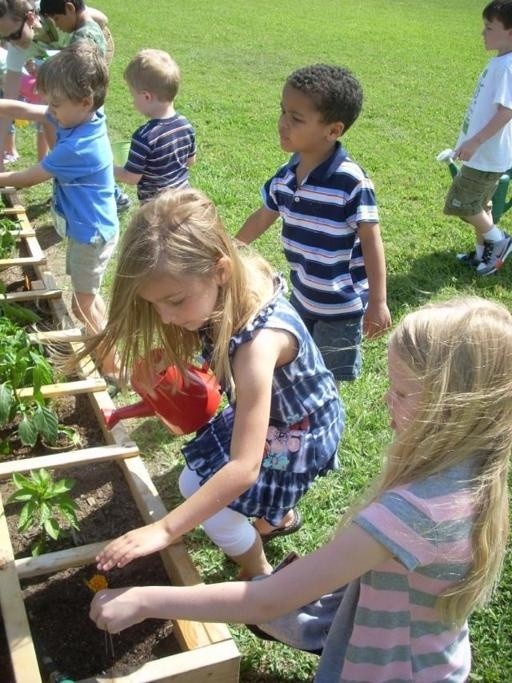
[457, 232, 512, 276]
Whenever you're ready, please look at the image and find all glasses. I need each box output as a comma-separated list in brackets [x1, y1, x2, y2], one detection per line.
[1, 15, 26, 42]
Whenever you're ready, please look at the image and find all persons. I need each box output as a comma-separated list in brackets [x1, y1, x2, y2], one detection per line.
[442, 0, 512, 276]
[111, 49, 195, 206]
[87, 295, 512, 683]
[232, 64, 392, 478]
[39, 187, 343, 585]
[1, 1, 127, 209]
[1, 45, 127, 387]
